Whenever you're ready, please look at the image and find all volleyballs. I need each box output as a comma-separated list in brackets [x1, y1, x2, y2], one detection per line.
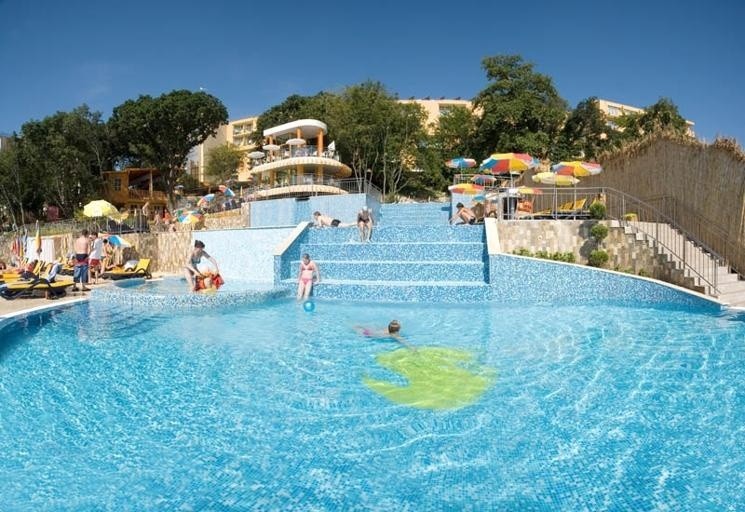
[304, 301, 314, 311]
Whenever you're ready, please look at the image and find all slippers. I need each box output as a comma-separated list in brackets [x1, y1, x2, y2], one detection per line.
[73, 287, 90, 291]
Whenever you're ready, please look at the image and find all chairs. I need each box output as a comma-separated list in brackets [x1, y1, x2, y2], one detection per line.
[0, 256, 152, 300]
[538, 194, 606, 220]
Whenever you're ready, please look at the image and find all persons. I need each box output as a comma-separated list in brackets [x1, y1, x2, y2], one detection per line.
[142, 201, 175, 231]
[353, 320, 414, 350]
[314, 212, 357, 228]
[69, 230, 115, 291]
[182, 241, 218, 292]
[357, 206, 373, 243]
[450, 203, 477, 224]
[296, 254, 320, 300]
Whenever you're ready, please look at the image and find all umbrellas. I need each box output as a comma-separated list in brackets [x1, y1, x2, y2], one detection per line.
[445, 153, 601, 219]
[12, 228, 27, 259]
[173, 184, 235, 228]
[287, 137, 305, 147]
[36, 219, 42, 279]
[247, 151, 264, 159]
[102, 235, 131, 249]
[263, 144, 281, 152]
[83, 199, 118, 232]
